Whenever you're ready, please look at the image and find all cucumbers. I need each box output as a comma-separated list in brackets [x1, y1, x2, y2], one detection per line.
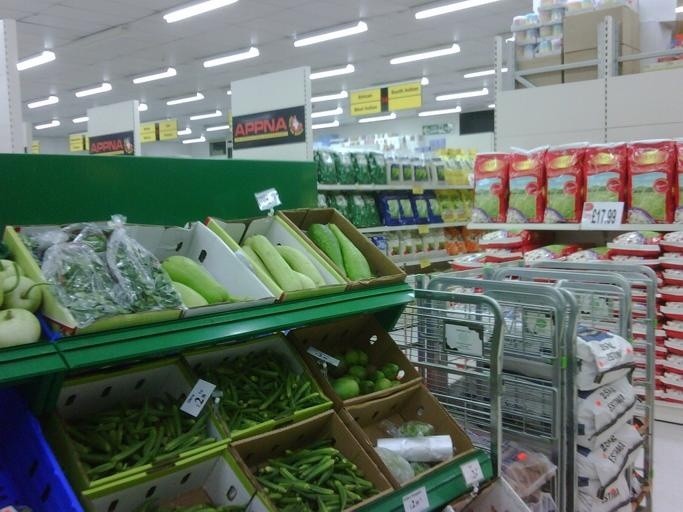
[242, 223, 372, 292]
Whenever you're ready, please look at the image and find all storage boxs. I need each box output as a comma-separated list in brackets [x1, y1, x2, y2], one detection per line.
[227, 409, 394, 511]
[338, 381, 475, 490]
[4, 223, 185, 339]
[511, 4, 642, 92]
[123, 220, 280, 319]
[79, 445, 275, 512]
[286, 317, 428, 411]
[1, 244, 56, 353]
[0, 382, 86, 512]
[47, 356, 234, 500]
[184, 335, 337, 445]
[440, 476, 532, 512]
[205, 215, 351, 304]
[276, 206, 408, 294]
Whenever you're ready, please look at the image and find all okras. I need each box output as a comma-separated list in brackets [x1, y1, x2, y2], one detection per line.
[192, 348, 328, 433]
[68, 388, 247, 512]
[253, 436, 381, 512]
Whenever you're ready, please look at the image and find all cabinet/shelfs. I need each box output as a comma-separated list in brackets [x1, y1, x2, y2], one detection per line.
[315, 182, 473, 333]
[456, 212, 681, 426]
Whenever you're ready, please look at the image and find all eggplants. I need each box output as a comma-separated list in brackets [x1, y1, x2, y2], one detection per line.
[163, 256, 229, 303]
[170, 281, 207, 309]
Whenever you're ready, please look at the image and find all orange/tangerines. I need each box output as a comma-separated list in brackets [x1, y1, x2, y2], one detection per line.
[327, 347, 401, 400]
